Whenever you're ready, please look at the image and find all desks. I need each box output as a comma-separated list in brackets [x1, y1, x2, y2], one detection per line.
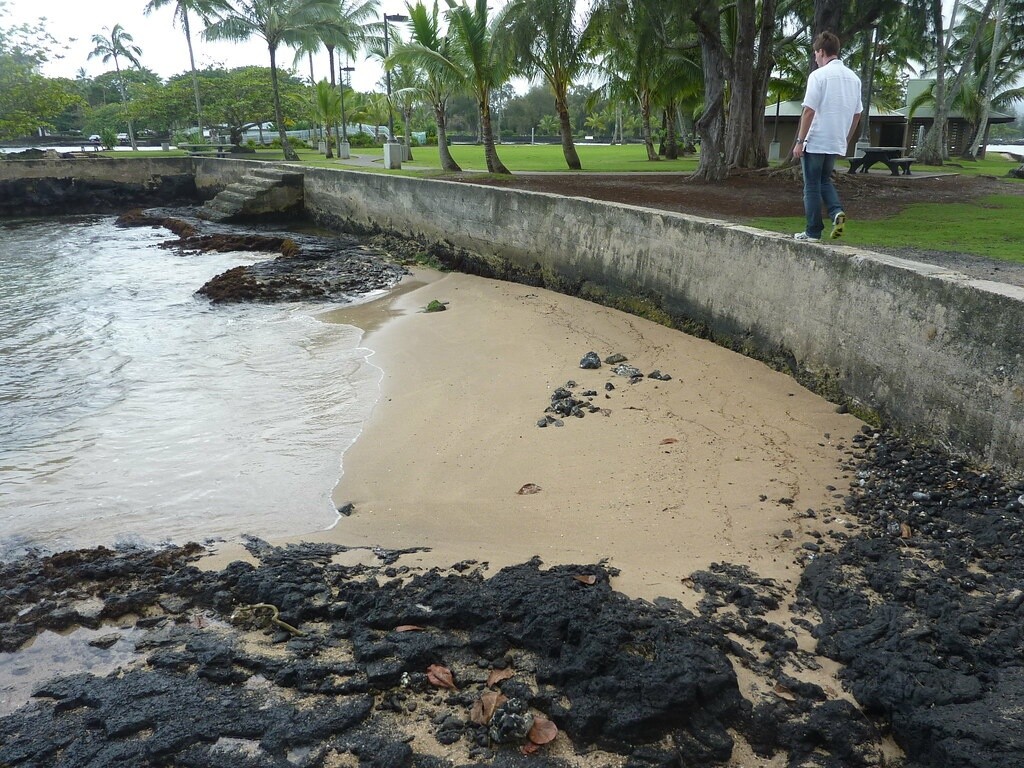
[178, 144, 236, 156]
[847, 147, 913, 176]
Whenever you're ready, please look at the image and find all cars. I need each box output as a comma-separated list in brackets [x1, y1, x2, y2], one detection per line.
[117, 133, 130, 142]
[88, 135, 101, 141]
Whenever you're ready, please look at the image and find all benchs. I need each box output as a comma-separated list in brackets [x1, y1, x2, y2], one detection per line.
[846, 157, 863, 160]
[890, 158, 917, 163]
[189, 152, 231, 158]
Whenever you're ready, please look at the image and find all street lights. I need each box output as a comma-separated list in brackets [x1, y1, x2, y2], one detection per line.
[339, 67, 355, 142]
[858, 24, 880, 142]
[384, 14, 408, 144]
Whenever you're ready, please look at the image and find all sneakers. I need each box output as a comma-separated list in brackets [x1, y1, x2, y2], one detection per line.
[830, 210, 846, 240]
[794, 231, 821, 243]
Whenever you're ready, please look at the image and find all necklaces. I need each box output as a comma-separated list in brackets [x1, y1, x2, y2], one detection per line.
[826, 58, 839, 64]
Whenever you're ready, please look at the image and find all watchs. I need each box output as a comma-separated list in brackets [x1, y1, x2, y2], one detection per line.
[797, 138, 804, 144]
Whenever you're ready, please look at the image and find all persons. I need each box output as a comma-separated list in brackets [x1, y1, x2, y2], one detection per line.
[793, 33, 863, 243]
[92, 137, 101, 151]
[211, 131, 224, 157]
